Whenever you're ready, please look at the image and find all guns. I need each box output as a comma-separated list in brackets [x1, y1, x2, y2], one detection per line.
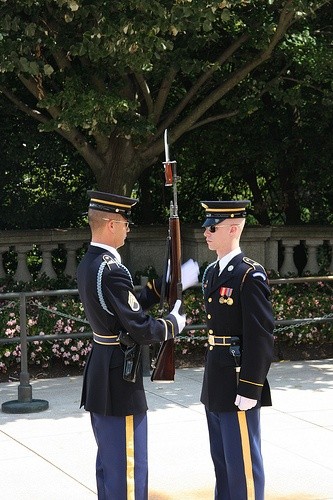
[150, 129, 184, 383]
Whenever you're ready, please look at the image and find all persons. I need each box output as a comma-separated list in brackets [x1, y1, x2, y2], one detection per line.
[78, 191, 200, 500]
[201, 201, 274, 500]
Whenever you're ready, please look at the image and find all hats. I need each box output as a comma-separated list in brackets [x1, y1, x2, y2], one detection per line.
[87, 189, 139, 223]
[199, 200, 250, 228]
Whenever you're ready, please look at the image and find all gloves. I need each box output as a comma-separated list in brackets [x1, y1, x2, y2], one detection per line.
[169, 299, 187, 333]
[166, 258, 200, 294]
[234, 394, 257, 410]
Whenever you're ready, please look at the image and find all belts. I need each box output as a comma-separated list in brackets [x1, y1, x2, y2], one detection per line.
[92, 332, 124, 346]
[208, 335, 233, 346]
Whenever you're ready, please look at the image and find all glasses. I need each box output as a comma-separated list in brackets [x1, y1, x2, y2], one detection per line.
[205, 224, 241, 233]
[102, 216, 129, 227]
